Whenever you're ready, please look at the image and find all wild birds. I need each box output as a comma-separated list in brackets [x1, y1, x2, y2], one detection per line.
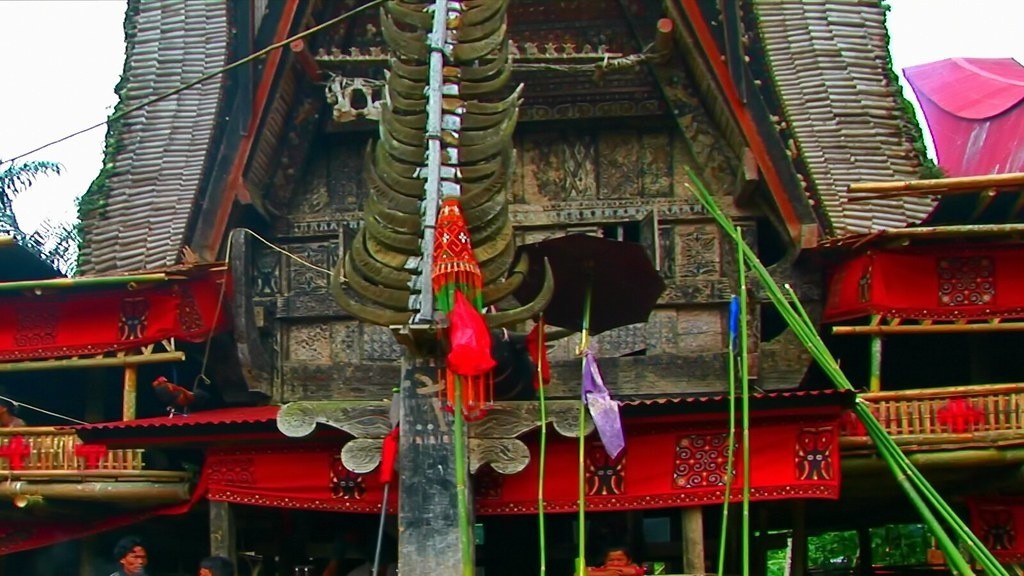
[151, 376, 195, 417]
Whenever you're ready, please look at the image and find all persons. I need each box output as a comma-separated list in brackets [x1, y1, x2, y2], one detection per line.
[199, 556, 234, 576]
[585, 548, 643, 576]
[110, 537, 147, 576]
[0, 401, 26, 427]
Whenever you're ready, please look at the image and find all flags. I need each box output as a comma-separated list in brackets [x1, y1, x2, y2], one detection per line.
[728, 297, 739, 352]
[525, 317, 550, 389]
[581, 350, 624, 459]
